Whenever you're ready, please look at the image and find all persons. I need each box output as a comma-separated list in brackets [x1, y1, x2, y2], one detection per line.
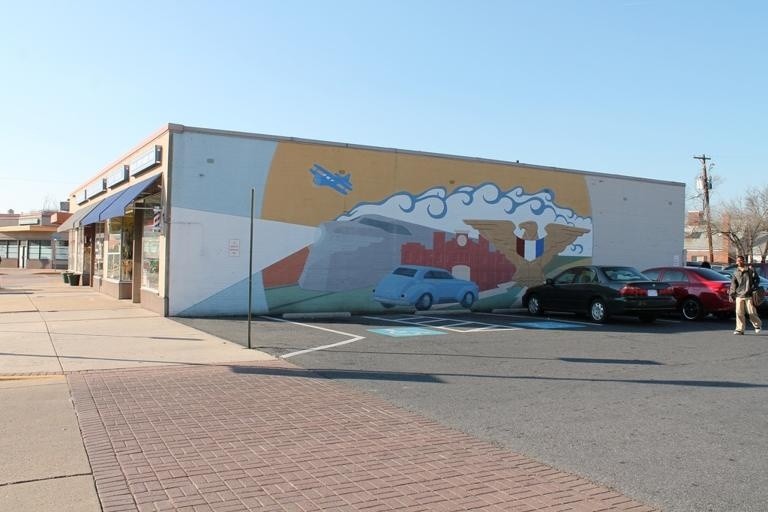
[728, 256, 762, 335]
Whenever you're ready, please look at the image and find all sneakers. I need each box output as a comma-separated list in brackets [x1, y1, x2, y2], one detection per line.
[754, 320, 761, 335]
[733, 329, 744, 335]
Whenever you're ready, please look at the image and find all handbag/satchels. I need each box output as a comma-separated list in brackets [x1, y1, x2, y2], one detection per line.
[752, 286, 766, 306]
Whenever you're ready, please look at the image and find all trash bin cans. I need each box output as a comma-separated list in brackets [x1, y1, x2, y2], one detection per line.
[62, 273, 80, 286]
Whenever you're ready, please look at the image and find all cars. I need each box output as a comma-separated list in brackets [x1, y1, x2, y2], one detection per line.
[372, 264, 479, 311]
[521, 262, 768, 324]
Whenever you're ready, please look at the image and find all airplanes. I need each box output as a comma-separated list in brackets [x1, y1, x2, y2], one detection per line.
[310, 162, 353, 196]
